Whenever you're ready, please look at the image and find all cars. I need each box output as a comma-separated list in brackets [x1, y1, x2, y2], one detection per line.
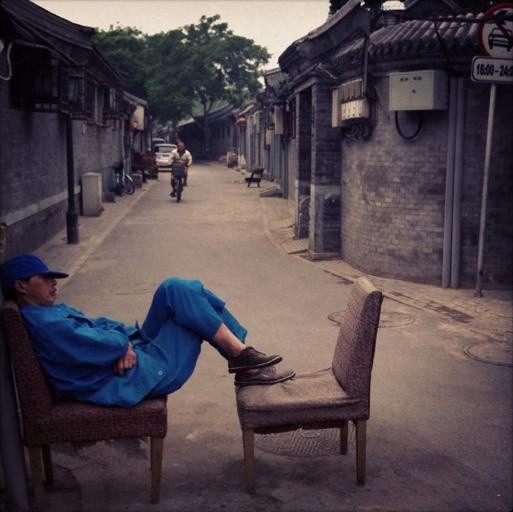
[146, 138, 177, 171]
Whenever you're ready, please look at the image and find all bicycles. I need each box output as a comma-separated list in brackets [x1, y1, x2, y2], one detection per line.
[169, 157, 188, 203]
[111, 156, 135, 198]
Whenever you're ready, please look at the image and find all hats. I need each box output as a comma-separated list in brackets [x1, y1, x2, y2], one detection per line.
[1, 254, 69, 285]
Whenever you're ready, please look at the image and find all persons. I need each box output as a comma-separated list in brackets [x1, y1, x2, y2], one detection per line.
[0, 253, 297, 411]
[166, 142, 192, 197]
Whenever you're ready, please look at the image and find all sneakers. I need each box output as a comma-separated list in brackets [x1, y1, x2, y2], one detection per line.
[182, 182, 188, 186]
[233, 366, 296, 386]
[228, 346, 283, 373]
[170, 189, 177, 195]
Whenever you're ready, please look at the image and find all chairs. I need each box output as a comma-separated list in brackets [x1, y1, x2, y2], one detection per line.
[224, 274, 383, 493]
[245, 167, 263, 187]
[2, 303, 167, 510]
